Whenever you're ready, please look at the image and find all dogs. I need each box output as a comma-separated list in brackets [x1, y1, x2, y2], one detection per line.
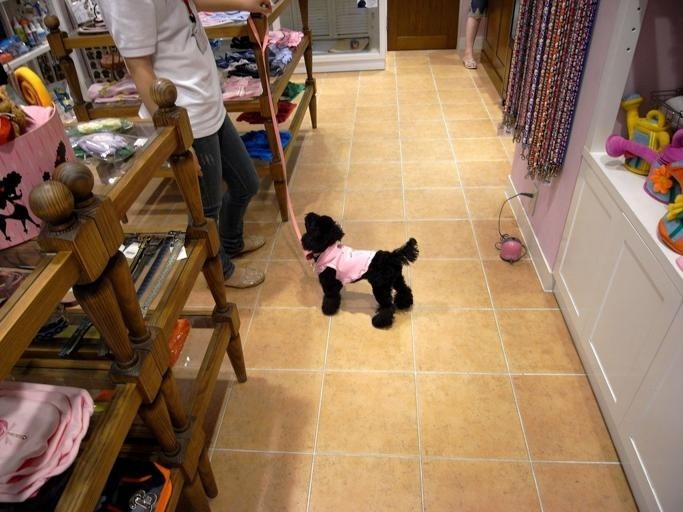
[300, 211, 419, 328]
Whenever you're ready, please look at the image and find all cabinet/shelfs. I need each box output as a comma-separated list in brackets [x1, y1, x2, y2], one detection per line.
[1, 79, 247, 512]
[44, 0, 317, 221]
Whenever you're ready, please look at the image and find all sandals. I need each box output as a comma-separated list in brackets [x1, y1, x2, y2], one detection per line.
[462, 56, 477, 69]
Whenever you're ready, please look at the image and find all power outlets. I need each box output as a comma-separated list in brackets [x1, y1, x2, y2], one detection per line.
[528, 184, 538, 216]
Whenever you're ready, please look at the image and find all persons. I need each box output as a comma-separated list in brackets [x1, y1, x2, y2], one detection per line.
[96, 0, 274, 289]
[461, 0, 491, 69]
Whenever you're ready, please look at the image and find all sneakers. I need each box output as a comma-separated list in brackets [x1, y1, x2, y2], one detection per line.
[224, 267, 265, 288]
[228, 235, 265, 258]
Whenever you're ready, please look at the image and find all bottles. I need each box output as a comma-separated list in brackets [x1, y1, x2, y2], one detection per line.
[12, 15, 50, 50]
